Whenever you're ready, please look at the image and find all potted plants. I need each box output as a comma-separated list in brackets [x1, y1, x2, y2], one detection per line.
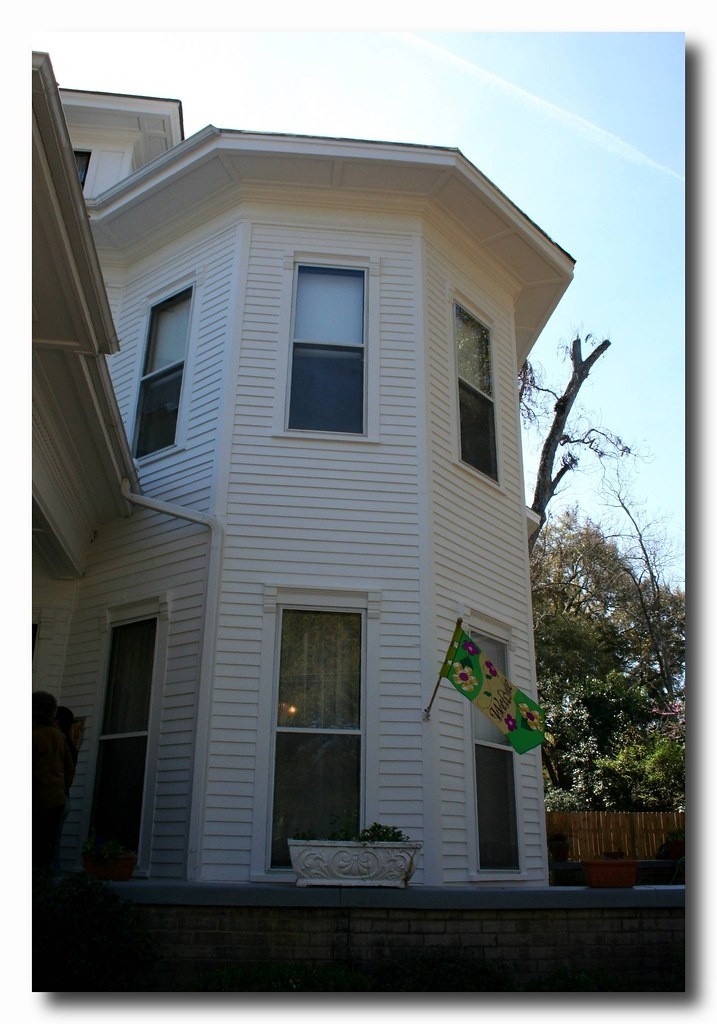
[286, 822, 425, 891]
[547, 828, 570, 863]
[657, 828, 685, 859]
[78, 825, 137, 883]
[584, 851, 637, 889]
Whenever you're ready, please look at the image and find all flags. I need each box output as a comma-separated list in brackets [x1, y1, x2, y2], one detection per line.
[438, 625, 546, 756]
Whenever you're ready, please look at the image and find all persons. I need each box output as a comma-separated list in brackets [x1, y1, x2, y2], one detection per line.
[46, 705, 77, 873]
[31, 690, 75, 866]
